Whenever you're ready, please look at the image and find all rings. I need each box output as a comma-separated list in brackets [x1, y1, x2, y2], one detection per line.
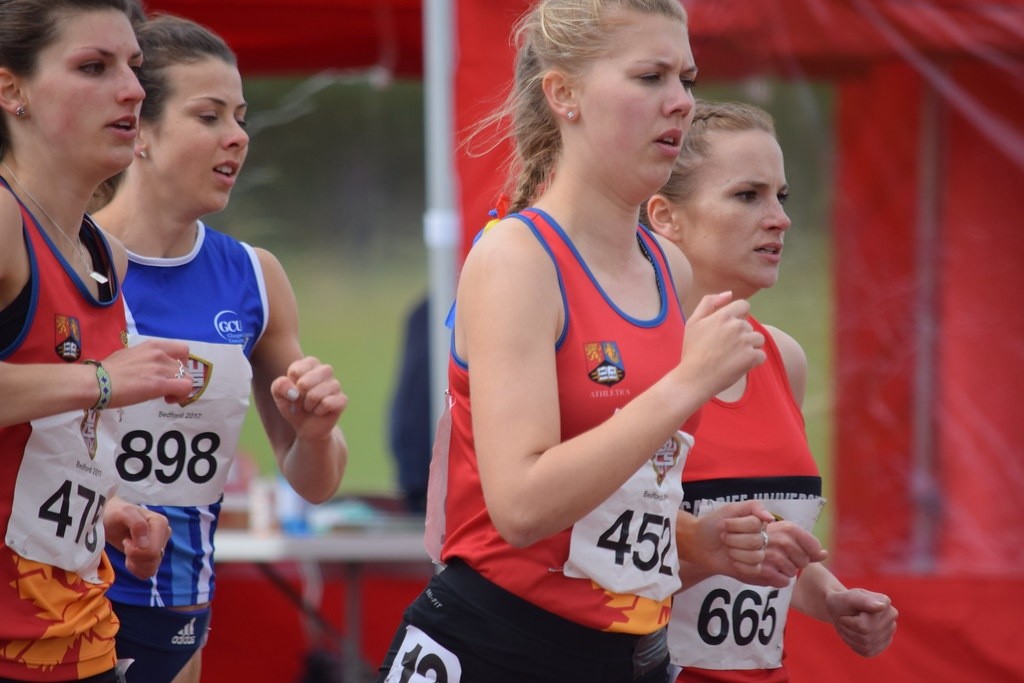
[175, 360, 185, 379]
[762, 531, 768, 550]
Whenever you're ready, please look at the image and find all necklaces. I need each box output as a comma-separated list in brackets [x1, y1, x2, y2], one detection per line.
[1, 162, 109, 284]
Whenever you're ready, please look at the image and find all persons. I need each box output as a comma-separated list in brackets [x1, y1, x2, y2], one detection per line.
[388, 299, 433, 514]
[639, 102, 900, 683]
[374, 0, 775, 683]
[0, 0, 192, 683]
[91, 16, 349, 683]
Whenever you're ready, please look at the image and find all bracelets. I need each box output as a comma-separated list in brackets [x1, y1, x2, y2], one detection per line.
[81, 359, 111, 411]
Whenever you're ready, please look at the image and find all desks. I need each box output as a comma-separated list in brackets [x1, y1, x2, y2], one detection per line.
[213, 529, 444, 683]
